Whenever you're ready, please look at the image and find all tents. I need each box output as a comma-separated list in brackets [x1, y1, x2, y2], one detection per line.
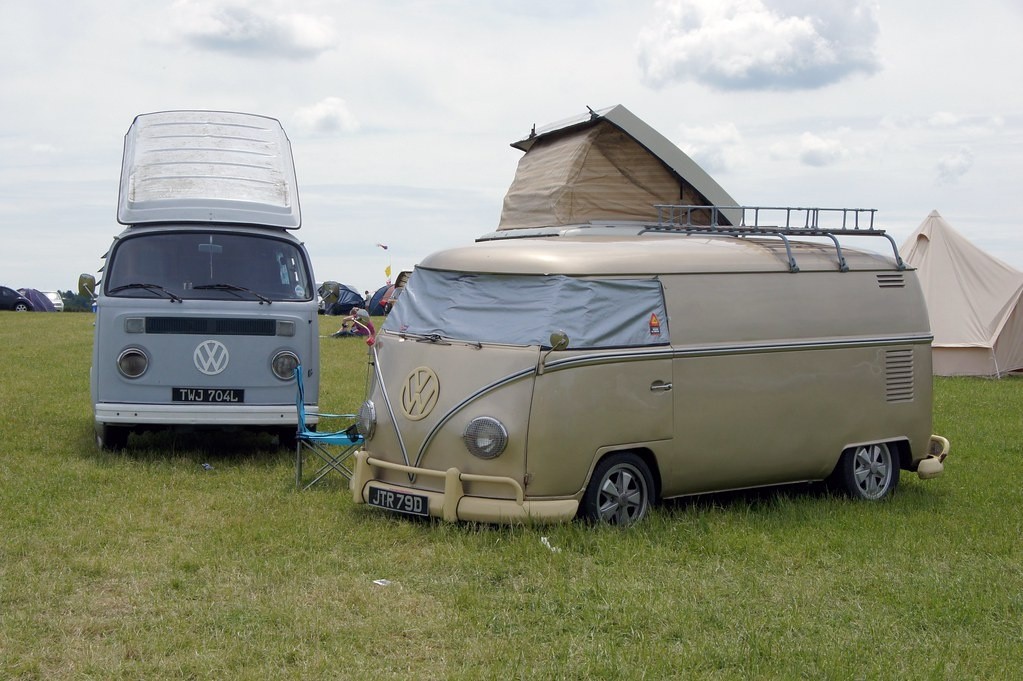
[891, 210, 1023, 377]
[17, 288, 57, 313]
[316, 281, 366, 315]
[368, 271, 412, 315]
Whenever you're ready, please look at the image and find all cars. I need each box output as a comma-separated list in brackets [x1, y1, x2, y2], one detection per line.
[0, 286, 35, 312]
[40, 291, 66, 312]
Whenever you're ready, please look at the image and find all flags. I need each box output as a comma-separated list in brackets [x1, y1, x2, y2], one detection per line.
[385, 265, 391, 277]
[377, 242, 389, 251]
[385, 278, 391, 285]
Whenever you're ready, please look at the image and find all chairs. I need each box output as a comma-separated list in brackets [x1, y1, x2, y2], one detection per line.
[293, 365, 364, 495]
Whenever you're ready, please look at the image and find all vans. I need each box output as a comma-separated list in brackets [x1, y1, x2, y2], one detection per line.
[352, 103, 950, 538]
[315, 281, 361, 315]
[78, 108, 340, 456]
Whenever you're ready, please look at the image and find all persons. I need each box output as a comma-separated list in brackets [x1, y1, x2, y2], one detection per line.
[365, 291, 371, 300]
[342, 307, 373, 336]
